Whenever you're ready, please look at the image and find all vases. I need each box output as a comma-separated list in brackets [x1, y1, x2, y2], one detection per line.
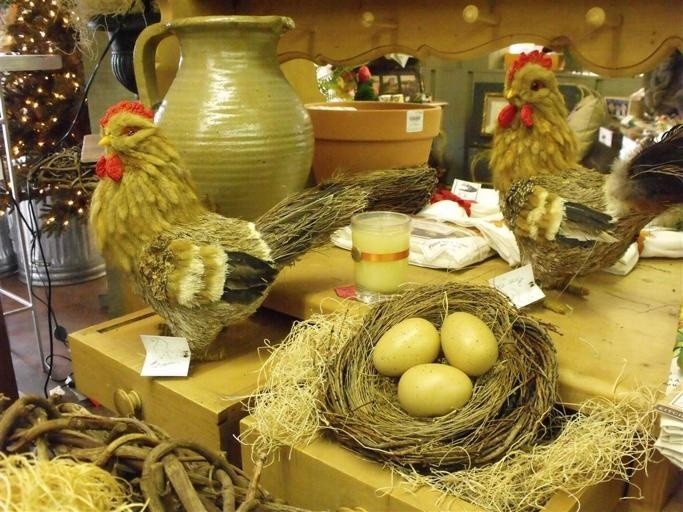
[131, 16, 315, 221]
[305, 100, 443, 185]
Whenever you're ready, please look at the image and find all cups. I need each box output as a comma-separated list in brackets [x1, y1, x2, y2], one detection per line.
[350, 211, 410, 304]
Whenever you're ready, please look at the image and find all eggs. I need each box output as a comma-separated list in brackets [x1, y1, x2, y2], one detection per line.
[440, 310, 499, 378]
[371, 318, 441, 377]
[396, 362, 472, 418]
